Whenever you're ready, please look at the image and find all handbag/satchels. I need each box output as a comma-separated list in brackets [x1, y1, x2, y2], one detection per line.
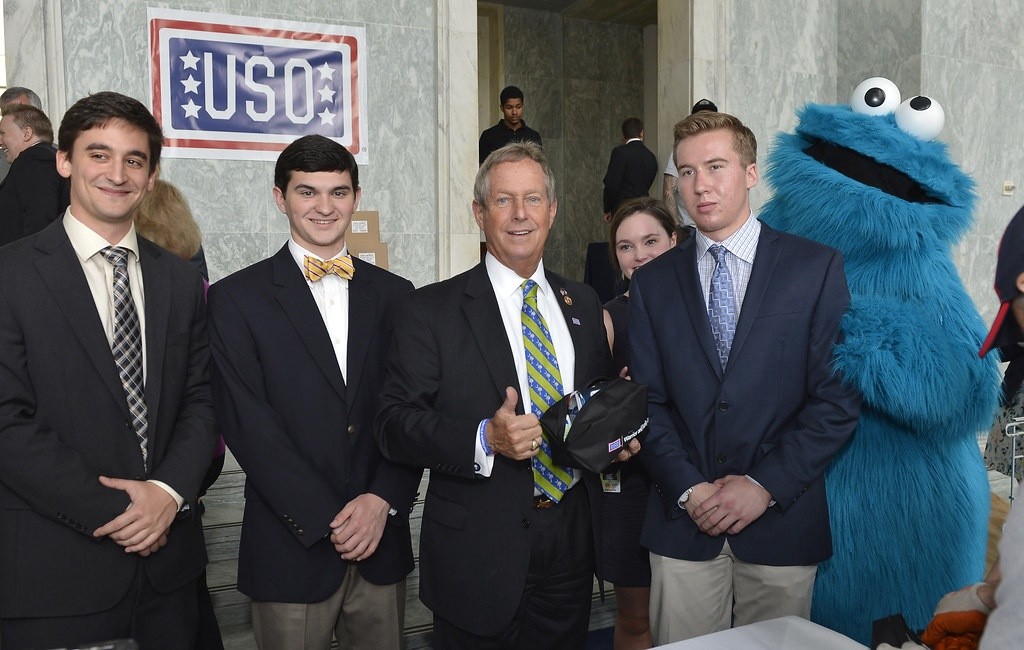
[539, 376, 651, 473]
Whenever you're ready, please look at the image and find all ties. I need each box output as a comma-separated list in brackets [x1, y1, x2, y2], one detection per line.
[98, 245, 149, 476]
[708, 244, 740, 374]
[521, 278, 574, 505]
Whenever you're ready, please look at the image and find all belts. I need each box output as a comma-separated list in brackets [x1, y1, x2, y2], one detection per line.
[530, 500, 554, 510]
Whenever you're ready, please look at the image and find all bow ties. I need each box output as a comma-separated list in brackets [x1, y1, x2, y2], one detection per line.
[304, 254, 355, 282]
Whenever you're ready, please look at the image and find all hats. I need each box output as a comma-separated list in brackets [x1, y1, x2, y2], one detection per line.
[978, 203, 1023, 358]
[691, 98, 717, 114]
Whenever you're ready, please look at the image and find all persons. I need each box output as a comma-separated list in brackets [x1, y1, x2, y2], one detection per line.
[921, 202, 1024, 650]
[663, 98, 718, 229]
[206, 134, 424, 650]
[0, 87, 70, 244]
[602, 117, 658, 223]
[479, 86, 543, 169]
[370, 143, 640, 650]
[594, 111, 859, 650]
[0, 92, 220, 650]
[133, 178, 225, 498]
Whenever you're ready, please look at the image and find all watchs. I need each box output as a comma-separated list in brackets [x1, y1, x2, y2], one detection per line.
[680, 486, 694, 507]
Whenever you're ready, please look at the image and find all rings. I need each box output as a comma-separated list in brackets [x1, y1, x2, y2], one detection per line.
[532, 439, 539, 451]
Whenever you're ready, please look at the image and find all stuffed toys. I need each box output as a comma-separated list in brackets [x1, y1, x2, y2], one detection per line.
[756, 77, 1004, 648]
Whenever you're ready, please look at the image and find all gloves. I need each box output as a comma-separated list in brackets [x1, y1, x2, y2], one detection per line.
[920, 582, 993, 650]
